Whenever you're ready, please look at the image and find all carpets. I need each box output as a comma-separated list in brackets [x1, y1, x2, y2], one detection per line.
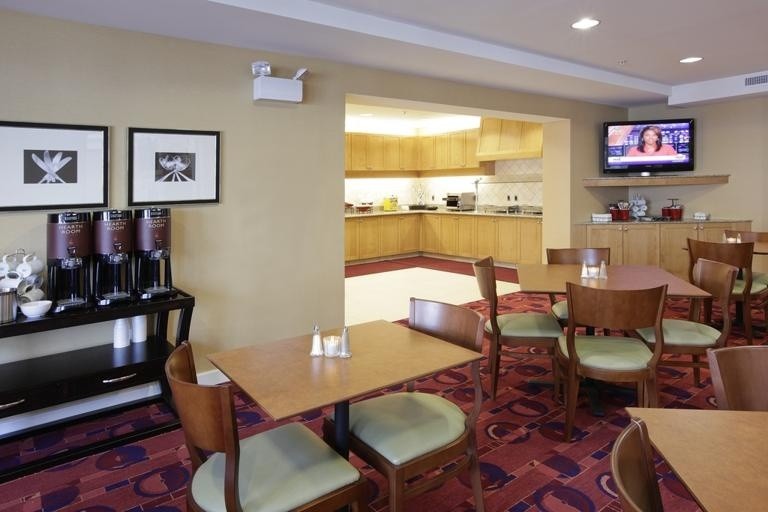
[344, 256, 519, 284]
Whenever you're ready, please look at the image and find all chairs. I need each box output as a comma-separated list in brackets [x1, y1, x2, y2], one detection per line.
[164, 341, 368, 512]
[322, 297, 485, 512]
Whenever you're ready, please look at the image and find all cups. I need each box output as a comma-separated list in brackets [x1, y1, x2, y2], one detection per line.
[323, 337, 340, 358]
[112, 342, 148, 370]
[587, 266, 599, 278]
[631, 197, 648, 220]
[662, 208, 682, 220]
[130, 313, 148, 344]
[0, 251, 46, 324]
[113, 319, 131, 349]
[610, 209, 630, 220]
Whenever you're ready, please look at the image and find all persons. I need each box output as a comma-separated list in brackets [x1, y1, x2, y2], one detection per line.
[625, 125, 677, 155]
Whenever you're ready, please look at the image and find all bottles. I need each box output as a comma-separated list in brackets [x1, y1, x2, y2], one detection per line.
[599, 259, 608, 279]
[580, 260, 588, 279]
[340, 324, 352, 360]
[582, 278, 607, 290]
[311, 355, 350, 380]
[309, 319, 324, 357]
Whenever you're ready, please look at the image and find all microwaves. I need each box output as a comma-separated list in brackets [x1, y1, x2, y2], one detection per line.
[443, 192, 476, 212]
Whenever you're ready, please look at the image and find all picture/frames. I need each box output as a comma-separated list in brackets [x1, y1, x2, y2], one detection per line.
[0, 120, 110, 211]
[128, 127, 220, 207]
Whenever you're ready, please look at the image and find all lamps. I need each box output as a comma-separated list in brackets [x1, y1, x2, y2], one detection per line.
[251, 60, 272, 77]
[293, 67, 308, 80]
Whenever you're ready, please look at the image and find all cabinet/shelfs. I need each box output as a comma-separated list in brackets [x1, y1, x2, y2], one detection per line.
[0, 287, 195, 485]
[345, 115, 544, 178]
[572, 220, 751, 282]
[344, 213, 542, 269]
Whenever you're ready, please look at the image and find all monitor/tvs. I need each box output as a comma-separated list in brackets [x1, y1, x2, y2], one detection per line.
[604, 119, 694, 173]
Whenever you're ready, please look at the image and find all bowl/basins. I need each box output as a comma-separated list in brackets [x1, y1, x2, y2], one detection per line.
[18, 301, 52, 318]
